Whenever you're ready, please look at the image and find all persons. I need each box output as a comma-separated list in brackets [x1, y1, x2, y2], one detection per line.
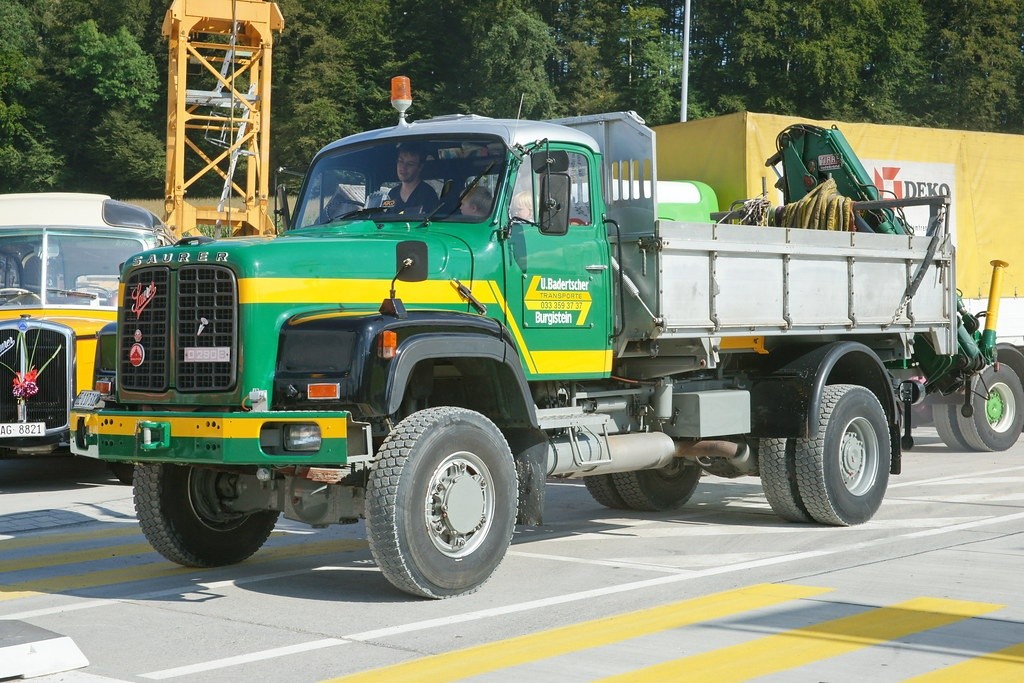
[509, 190, 540, 224]
[457, 186, 497, 216]
[383, 142, 442, 216]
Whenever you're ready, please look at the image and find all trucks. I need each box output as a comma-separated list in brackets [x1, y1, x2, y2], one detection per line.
[72, 73, 959, 601]
[571, 110, 1024, 453]
[1, 191, 175, 486]
